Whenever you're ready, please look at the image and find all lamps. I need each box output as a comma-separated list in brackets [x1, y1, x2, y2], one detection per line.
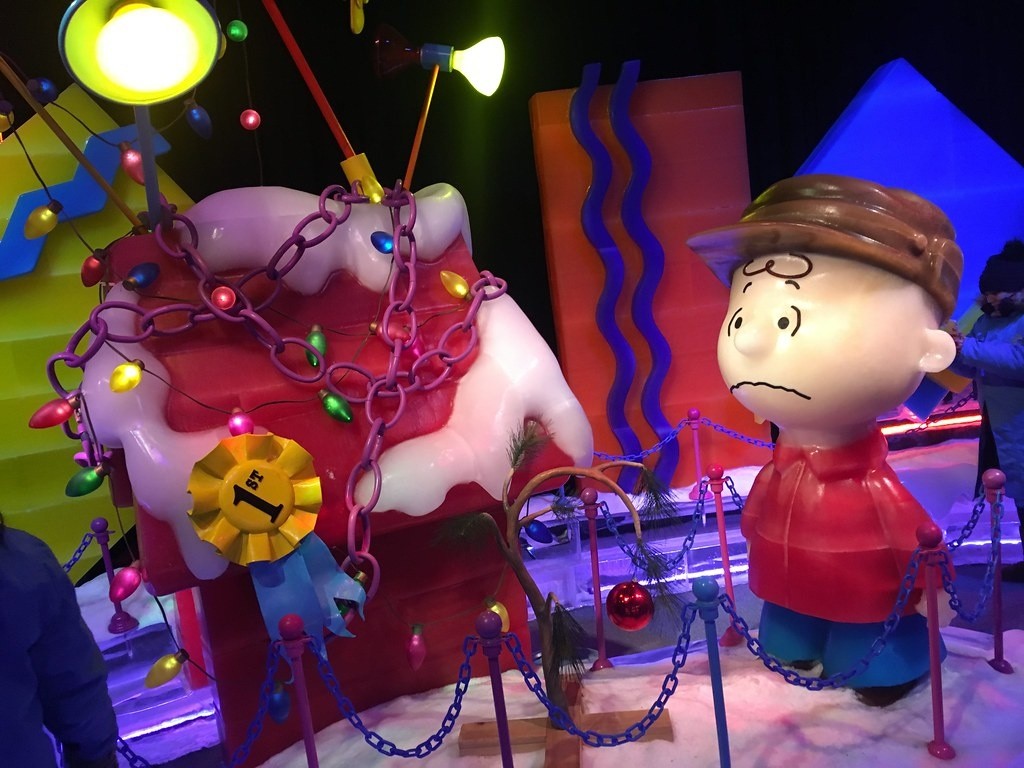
[367, 21, 509, 100]
[56, 0, 225, 109]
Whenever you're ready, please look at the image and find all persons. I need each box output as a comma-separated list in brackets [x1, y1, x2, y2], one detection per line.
[685, 174, 965, 707]
[949, 240, 1024, 582]
[0, 511, 119, 768]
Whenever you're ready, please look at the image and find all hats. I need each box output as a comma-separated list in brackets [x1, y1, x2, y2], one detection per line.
[979, 239, 1024, 294]
